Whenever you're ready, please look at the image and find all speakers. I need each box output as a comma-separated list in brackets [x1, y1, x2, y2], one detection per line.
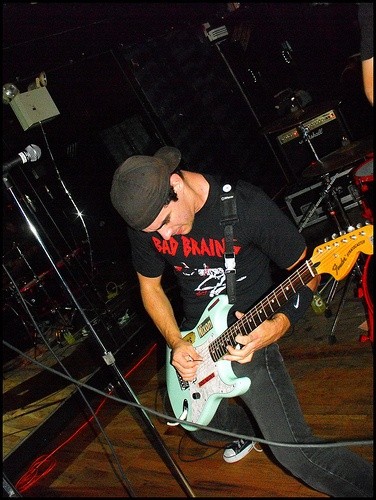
[117, 25, 293, 199]
[264, 105, 352, 185]
[214, 19, 307, 127]
[20, 52, 172, 227]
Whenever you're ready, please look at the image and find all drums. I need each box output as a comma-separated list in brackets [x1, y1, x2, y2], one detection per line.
[54, 247, 101, 298]
[17, 269, 69, 319]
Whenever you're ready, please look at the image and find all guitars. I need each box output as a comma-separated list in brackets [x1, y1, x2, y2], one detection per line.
[165, 220, 374, 433]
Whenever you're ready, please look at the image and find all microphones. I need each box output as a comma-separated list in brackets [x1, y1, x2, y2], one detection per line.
[0, 143, 44, 175]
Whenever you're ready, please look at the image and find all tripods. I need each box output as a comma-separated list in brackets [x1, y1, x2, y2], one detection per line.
[2, 236, 103, 361]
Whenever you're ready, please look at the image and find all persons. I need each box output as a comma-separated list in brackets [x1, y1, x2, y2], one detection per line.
[109, 145, 376, 500]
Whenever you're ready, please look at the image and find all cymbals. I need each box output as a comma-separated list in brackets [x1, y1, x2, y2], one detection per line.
[1, 236, 32, 262]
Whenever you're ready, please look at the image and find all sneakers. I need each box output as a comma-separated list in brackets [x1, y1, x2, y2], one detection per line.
[223, 438, 263, 463]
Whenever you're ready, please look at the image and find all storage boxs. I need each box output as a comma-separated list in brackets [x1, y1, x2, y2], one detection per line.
[285, 166, 364, 229]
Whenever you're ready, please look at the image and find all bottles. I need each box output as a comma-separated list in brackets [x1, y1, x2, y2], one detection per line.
[63, 328, 75, 345]
[310, 293, 328, 314]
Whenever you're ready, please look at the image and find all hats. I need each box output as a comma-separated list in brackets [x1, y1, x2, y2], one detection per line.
[109, 146, 182, 229]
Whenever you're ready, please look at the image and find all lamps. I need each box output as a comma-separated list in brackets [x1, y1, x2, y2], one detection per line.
[3, 72, 60, 132]
